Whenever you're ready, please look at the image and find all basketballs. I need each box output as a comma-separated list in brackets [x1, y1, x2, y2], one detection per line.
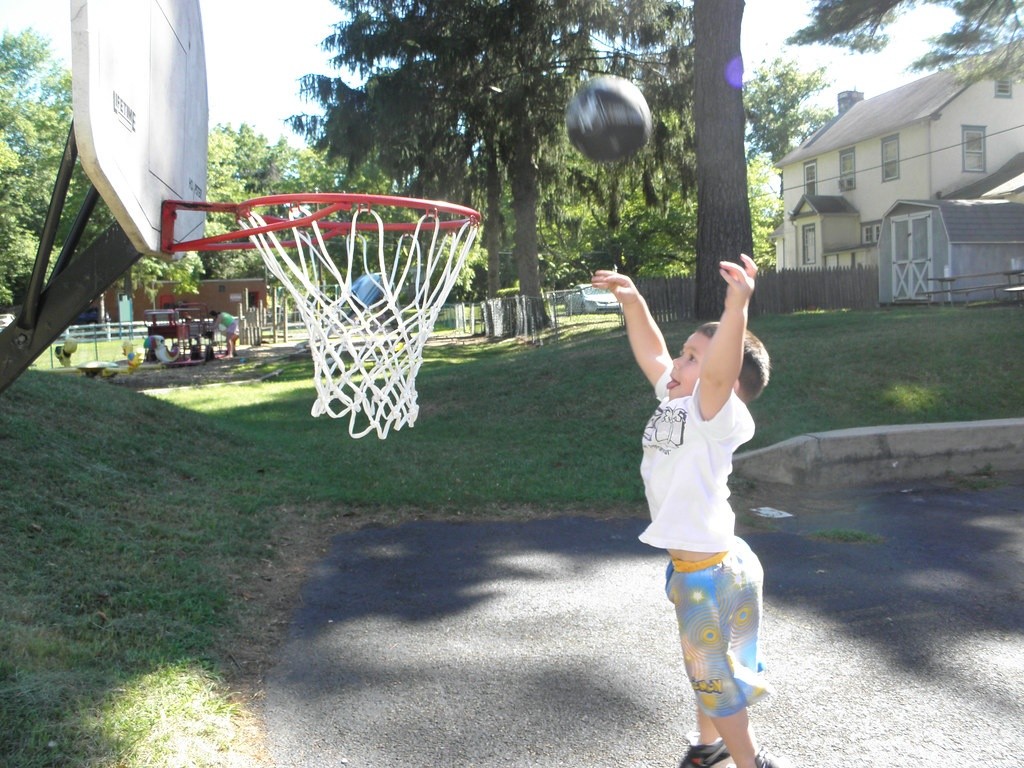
[562, 74, 655, 165]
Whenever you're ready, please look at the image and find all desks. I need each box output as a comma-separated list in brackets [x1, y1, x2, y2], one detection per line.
[143, 303, 215, 359]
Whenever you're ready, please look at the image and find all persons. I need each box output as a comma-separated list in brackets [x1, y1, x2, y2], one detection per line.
[591, 254, 778, 767]
[206, 310, 239, 359]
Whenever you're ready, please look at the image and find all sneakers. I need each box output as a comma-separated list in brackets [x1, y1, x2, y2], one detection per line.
[679, 731, 781, 767]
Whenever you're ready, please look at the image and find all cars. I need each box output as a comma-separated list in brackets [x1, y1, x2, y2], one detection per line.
[0, 313, 16, 333]
[564, 283, 623, 315]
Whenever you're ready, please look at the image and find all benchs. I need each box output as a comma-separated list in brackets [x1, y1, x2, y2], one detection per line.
[918, 269, 1023, 308]
